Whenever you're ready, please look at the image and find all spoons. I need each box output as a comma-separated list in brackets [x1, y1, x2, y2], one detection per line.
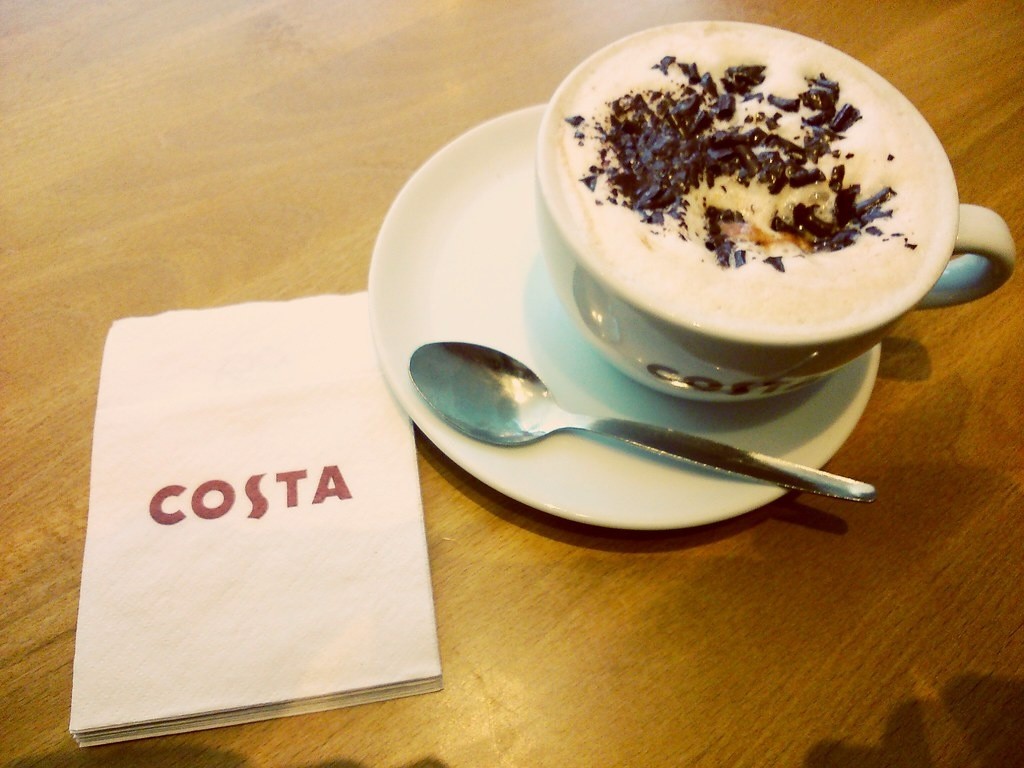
[409, 341, 876, 504]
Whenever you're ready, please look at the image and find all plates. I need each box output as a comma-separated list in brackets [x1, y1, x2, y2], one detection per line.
[368, 103, 881, 529]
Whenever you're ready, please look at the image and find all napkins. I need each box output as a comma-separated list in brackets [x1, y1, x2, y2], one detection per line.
[69, 290, 443, 749]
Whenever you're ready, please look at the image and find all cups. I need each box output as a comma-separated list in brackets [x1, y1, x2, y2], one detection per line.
[536, 19, 1016, 402]
